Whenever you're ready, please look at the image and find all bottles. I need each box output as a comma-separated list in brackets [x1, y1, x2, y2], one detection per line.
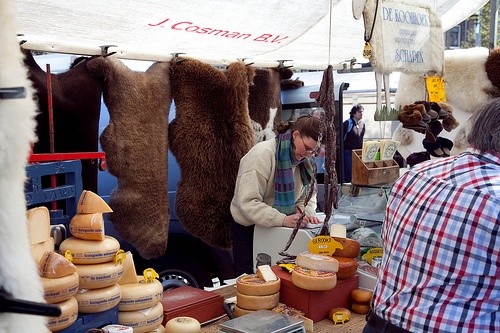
[343, 57, 356, 69]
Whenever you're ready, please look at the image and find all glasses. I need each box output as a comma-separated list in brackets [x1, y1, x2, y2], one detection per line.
[299, 137, 317, 156]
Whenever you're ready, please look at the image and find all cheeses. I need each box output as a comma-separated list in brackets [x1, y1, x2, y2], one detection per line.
[292, 253, 339, 291]
[233, 264, 281, 316]
[24, 190, 201, 333]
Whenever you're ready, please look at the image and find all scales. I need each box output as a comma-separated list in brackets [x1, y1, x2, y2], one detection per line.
[216, 309, 306, 333]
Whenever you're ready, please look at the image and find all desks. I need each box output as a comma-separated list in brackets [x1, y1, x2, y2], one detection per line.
[200, 283, 370, 333]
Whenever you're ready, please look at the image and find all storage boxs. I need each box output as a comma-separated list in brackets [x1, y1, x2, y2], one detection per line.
[162, 286, 226, 328]
[269, 265, 360, 322]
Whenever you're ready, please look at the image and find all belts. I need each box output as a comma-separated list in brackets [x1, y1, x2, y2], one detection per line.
[366, 313, 408, 333]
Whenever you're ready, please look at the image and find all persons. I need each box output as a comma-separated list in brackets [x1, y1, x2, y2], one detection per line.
[230, 114, 334, 277]
[342, 103, 365, 184]
[359, 95, 500, 333]
